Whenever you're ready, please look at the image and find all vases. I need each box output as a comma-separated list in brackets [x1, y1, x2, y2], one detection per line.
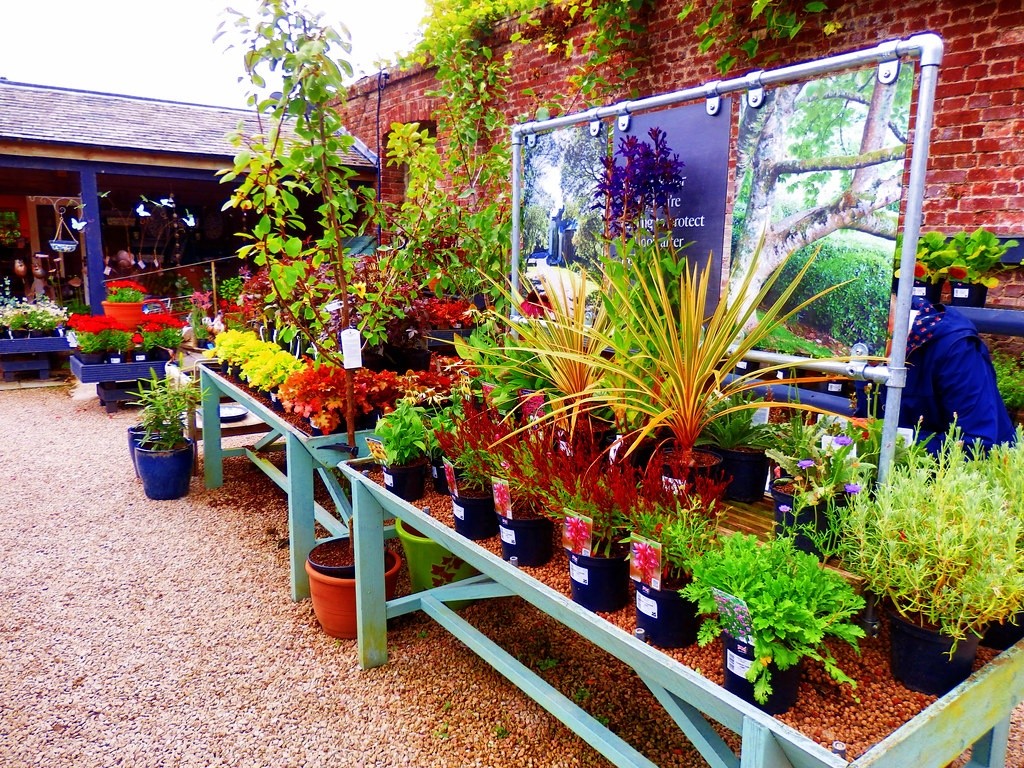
[102, 301, 145, 313]
[49, 241, 78, 252]
[75, 350, 176, 365]
[197, 339, 209, 348]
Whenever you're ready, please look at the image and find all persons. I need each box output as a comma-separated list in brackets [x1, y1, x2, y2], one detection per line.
[856, 293, 1018, 480]
[111, 250, 132, 276]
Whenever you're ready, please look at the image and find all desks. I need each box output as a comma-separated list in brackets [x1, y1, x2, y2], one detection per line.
[335, 459, 1024, 768]
[0, 327, 75, 381]
[70, 347, 189, 412]
[198, 362, 398, 602]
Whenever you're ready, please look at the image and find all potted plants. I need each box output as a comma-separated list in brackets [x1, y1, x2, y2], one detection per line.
[126, 0, 1024, 719]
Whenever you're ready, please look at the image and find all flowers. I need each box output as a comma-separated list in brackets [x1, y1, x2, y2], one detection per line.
[0, 276, 73, 332]
[106, 280, 148, 302]
[67, 313, 190, 352]
[189, 292, 213, 338]
